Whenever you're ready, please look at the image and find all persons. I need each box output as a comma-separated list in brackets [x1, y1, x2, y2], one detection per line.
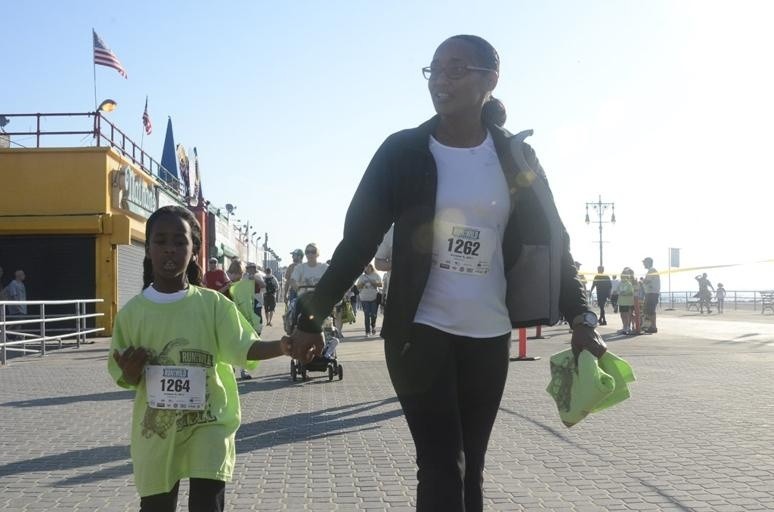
[199, 240, 338, 375]
[717, 283, 726, 316]
[576, 258, 663, 335]
[343, 224, 392, 341]
[285, 35, 607, 510]
[105, 205, 291, 511]
[693, 271, 714, 311]
[2, 265, 29, 337]
[0, 114, 11, 151]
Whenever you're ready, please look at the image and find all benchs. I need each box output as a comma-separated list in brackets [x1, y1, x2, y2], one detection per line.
[687, 301, 717, 312]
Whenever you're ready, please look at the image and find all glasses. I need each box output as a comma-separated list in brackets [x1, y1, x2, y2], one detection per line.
[421, 63, 501, 80]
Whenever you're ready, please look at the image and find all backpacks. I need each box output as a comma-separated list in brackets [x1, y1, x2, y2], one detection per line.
[265, 277, 274, 294]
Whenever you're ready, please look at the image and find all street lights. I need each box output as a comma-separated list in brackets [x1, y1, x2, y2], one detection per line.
[583, 193, 615, 266]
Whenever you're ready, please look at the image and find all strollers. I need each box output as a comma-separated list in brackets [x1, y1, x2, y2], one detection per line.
[281, 285, 345, 384]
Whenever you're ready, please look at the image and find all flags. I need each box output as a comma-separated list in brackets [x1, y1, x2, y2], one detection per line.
[157, 117, 203, 203]
[141, 100, 155, 133]
[94, 31, 127, 83]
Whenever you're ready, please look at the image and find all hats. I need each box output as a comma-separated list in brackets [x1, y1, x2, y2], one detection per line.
[290, 249, 304, 256]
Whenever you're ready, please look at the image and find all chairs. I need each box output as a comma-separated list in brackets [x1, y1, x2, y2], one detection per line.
[759, 291, 774, 315]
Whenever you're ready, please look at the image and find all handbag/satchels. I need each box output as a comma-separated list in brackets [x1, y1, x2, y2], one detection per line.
[376, 292, 382, 305]
